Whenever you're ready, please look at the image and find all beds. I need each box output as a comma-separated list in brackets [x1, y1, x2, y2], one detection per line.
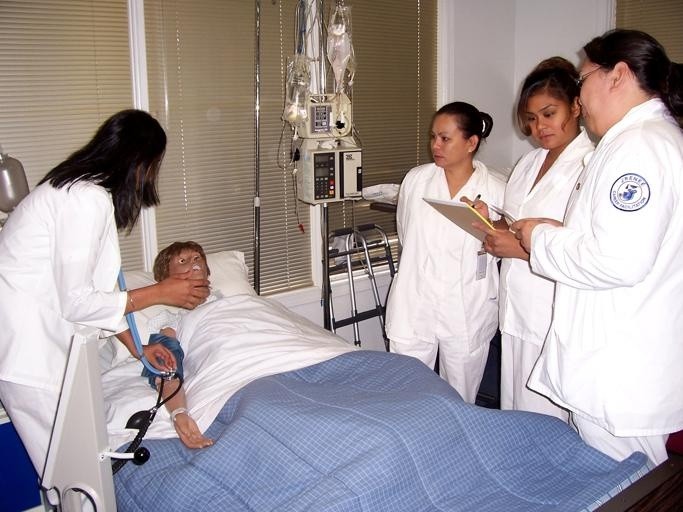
[99, 293, 683, 512]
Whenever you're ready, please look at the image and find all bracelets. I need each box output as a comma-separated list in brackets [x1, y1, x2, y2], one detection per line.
[126, 290, 137, 310]
[170, 407, 190, 428]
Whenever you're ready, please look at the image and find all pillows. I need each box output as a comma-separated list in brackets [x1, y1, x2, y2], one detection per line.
[117, 250, 256, 343]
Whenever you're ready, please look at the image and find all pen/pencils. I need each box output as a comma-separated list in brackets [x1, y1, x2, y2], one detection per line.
[471, 194, 481, 208]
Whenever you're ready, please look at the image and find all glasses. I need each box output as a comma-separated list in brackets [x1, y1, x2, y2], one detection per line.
[574, 64, 604, 89]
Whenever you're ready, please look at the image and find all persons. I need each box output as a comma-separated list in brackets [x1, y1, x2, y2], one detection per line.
[385, 102, 507, 406]
[509, 29, 682, 468]
[138, 241, 211, 450]
[0, 109, 211, 481]
[460, 55, 594, 423]
